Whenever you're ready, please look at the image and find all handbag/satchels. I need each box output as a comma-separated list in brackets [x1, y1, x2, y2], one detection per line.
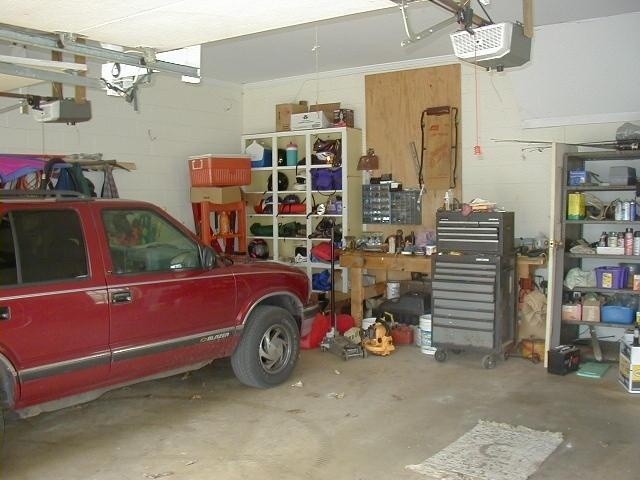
[310, 167, 342, 190]
[356, 147, 379, 171]
[314, 139, 341, 166]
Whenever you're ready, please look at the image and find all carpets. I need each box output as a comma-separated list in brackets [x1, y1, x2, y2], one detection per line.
[405, 416, 564, 480]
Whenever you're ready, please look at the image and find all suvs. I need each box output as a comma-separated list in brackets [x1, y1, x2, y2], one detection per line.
[0, 186, 314, 425]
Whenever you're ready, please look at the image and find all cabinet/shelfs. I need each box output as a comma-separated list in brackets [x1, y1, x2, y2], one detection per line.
[201, 202, 246, 255]
[239, 126, 363, 293]
[542, 141, 640, 371]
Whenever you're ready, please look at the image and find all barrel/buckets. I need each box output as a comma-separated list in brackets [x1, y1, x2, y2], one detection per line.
[418, 314, 439, 354]
[387, 282, 400, 299]
[362, 317, 375, 331]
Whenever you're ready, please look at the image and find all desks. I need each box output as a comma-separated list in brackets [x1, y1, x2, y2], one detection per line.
[339, 249, 431, 327]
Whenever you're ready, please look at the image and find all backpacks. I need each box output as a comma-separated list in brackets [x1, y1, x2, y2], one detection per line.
[44, 157, 98, 198]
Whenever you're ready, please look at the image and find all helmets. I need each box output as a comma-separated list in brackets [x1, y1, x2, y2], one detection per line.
[248, 238, 269, 259]
[267, 172, 288, 191]
[265, 195, 300, 205]
[293, 170, 307, 191]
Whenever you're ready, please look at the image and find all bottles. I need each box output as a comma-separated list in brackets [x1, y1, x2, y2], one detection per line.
[598, 227, 640, 256]
[286, 142, 298, 166]
[389, 236, 396, 253]
[615, 200, 636, 222]
[443, 191, 453, 211]
[634, 311, 640, 346]
[410, 231, 416, 244]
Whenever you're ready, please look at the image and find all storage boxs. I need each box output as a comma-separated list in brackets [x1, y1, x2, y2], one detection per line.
[569, 170, 591, 185]
[581, 297, 612, 323]
[560, 300, 581, 321]
[275, 101, 308, 131]
[547, 345, 580, 375]
[289, 111, 334, 131]
[594, 266, 628, 289]
[245, 139, 272, 168]
[189, 186, 242, 205]
[187, 153, 252, 187]
[618, 333, 640, 394]
[607, 166, 636, 185]
[600, 298, 639, 324]
